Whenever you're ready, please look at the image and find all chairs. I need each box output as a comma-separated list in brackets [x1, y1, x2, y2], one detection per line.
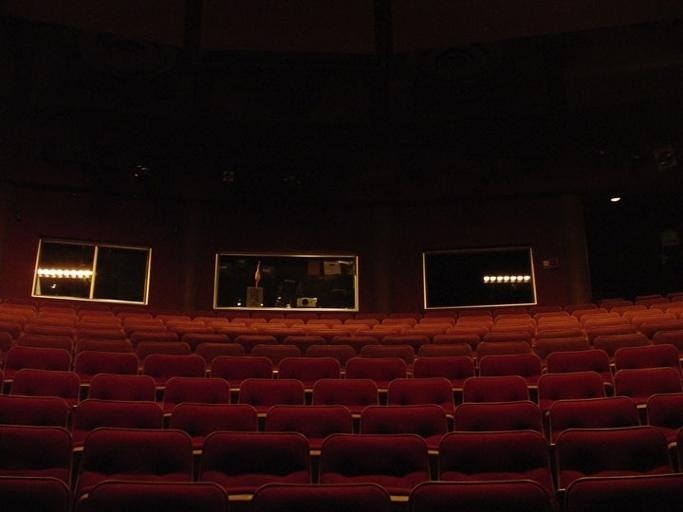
[0, 291, 682, 511]
